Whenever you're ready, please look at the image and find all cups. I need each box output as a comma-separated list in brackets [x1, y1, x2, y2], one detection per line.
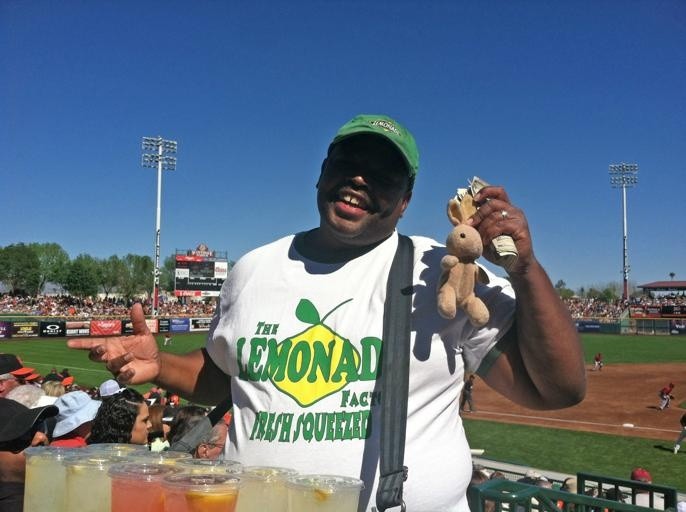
[21, 441, 365, 511]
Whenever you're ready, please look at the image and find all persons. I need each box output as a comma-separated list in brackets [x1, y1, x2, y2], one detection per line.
[0, 294, 217, 316]
[559, 292, 686, 325]
[469, 463, 686, 512]
[672, 413, 686, 456]
[66, 112, 587, 512]
[657, 381, 675, 411]
[460, 373, 478, 418]
[0, 352, 235, 512]
[591, 352, 603, 371]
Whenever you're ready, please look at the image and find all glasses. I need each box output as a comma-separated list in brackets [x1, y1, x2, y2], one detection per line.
[8, 419, 49, 453]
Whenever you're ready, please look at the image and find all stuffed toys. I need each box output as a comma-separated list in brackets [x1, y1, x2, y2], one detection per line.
[434, 195, 490, 329]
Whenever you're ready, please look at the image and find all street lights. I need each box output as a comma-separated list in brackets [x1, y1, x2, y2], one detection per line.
[138, 135, 179, 318]
[607, 162, 641, 300]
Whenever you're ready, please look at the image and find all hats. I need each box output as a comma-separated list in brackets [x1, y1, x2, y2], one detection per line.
[1, 397, 60, 444]
[3, 384, 59, 410]
[51, 390, 103, 440]
[0, 352, 42, 383]
[327, 113, 421, 179]
[40, 371, 75, 387]
[99, 379, 128, 399]
